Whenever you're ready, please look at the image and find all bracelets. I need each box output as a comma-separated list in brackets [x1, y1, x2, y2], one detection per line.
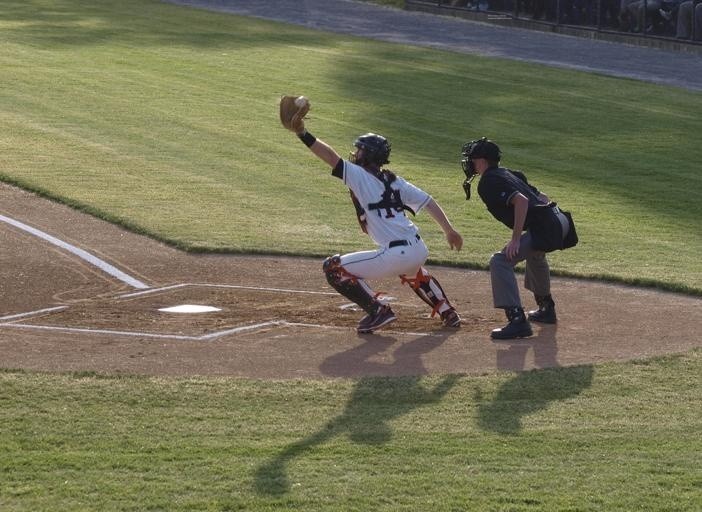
[298, 130, 317, 149]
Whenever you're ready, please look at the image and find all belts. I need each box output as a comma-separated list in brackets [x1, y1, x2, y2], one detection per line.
[383, 234, 421, 247]
[553, 207, 561, 214]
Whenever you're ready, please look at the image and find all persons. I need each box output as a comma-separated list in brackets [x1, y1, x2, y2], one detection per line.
[279, 94, 463, 335]
[441, 0, 702, 43]
[459, 137, 579, 340]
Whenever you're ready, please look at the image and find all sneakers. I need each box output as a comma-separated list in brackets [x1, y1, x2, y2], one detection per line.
[442, 309, 462, 328]
[461, 137, 503, 162]
[491, 319, 534, 340]
[527, 306, 557, 326]
[355, 306, 397, 334]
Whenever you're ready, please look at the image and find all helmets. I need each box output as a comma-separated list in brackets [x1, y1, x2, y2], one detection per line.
[351, 131, 390, 167]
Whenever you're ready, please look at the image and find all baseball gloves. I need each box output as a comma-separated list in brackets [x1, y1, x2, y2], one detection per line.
[280, 95, 310, 132]
